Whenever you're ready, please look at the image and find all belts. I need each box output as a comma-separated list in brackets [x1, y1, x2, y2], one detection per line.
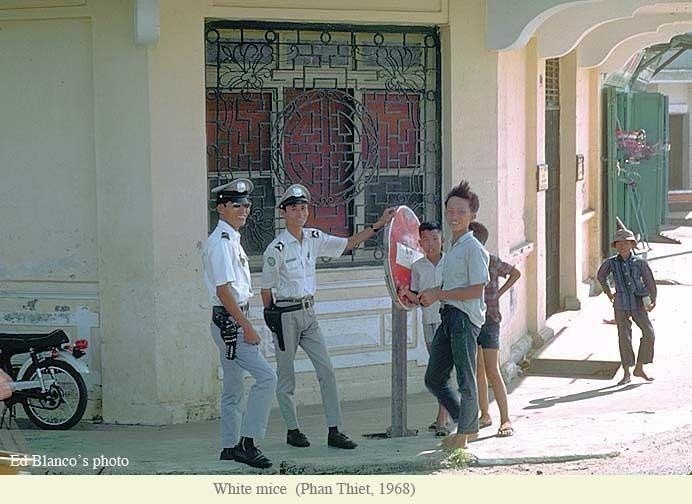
[214, 303, 250, 312]
[276, 296, 313, 304]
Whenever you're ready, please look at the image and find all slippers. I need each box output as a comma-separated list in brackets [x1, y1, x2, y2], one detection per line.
[435, 428, 449, 436]
[479, 423, 492, 430]
[498, 425, 515, 437]
[429, 422, 438, 431]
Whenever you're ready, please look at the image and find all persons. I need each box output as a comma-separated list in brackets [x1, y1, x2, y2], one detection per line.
[203, 178, 278, 467]
[469, 221, 522, 437]
[398, 221, 456, 437]
[417, 179, 490, 451]
[260, 184, 396, 449]
[597, 229, 656, 386]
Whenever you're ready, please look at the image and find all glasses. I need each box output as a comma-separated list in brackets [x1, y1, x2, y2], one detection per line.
[232, 203, 250, 208]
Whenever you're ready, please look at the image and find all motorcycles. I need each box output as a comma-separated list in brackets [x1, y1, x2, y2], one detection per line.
[0, 327, 91, 430]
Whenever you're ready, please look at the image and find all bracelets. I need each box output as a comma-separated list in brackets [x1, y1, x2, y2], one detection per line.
[370, 224, 379, 234]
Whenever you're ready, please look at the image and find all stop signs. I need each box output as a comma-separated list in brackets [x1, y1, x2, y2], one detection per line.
[386, 205, 428, 312]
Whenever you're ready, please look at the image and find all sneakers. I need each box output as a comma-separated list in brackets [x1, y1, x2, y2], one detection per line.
[328, 431, 357, 449]
[287, 432, 310, 448]
[220, 444, 273, 468]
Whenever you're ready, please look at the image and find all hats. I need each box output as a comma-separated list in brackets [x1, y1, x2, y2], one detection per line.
[276, 184, 310, 208]
[612, 229, 638, 249]
[212, 178, 253, 203]
